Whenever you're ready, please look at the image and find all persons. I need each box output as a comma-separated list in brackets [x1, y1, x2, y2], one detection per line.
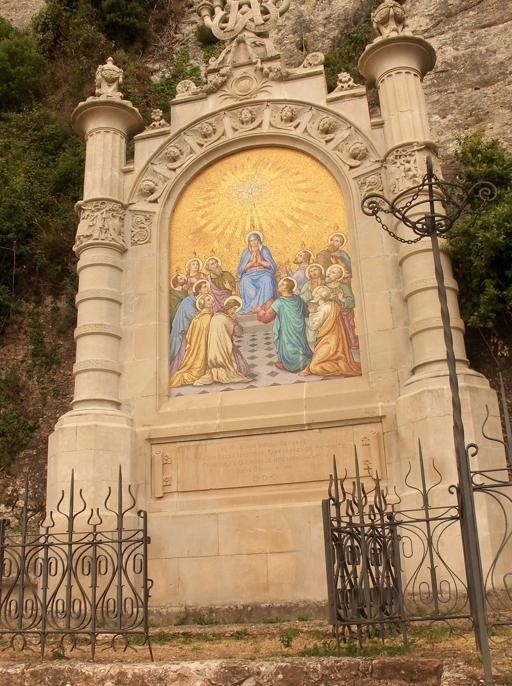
[168, 231, 361, 387]
